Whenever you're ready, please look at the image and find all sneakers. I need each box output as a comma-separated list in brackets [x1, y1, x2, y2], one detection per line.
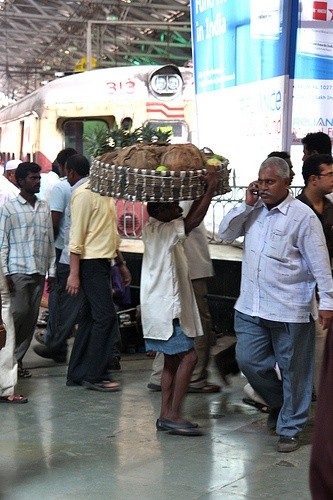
[267, 407, 281, 428]
[277, 435, 300, 452]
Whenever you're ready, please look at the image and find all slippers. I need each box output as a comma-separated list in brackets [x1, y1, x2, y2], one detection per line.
[242, 398, 268, 413]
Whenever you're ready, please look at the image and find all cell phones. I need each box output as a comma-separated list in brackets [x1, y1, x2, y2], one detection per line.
[252, 184, 259, 195]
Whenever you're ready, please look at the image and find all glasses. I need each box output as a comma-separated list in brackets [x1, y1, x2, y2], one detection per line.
[316, 171, 333, 178]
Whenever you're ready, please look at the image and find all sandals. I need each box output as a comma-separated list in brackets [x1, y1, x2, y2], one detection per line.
[81, 376, 121, 392]
[0, 393, 28, 404]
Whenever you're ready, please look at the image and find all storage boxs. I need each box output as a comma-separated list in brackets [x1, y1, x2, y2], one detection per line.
[89, 147, 232, 202]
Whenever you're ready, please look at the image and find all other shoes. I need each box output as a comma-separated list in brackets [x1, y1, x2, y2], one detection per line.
[35, 330, 48, 344]
[34, 344, 67, 362]
[188, 384, 221, 393]
[18, 367, 31, 378]
[155, 418, 204, 437]
[147, 383, 162, 391]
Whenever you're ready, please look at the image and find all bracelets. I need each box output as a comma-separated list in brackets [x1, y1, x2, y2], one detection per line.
[114, 261, 126, 268]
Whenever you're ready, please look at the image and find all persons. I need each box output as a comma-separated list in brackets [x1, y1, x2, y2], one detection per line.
[217, 156, 333, 453]
[35, 147, 78, 346]
[0, 255, 28, 404]
[33, 154, 121, 371]
[242, 156, 333, 412]
[36, 159, 63, 328]
[63, 178, 132, 393]
[146, 200, 221, 394]
[0, 158, 24, 218]
[267, 152, 296, 190]
[301, 132, 333, 204]
[0, 162, 57, 379]
[140, 164, 218, 436]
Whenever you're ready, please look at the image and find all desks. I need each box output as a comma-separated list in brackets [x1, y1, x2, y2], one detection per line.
[118, 239, 243, 336]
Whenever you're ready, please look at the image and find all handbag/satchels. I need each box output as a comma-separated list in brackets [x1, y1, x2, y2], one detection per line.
[111, 261, 132, 308]
